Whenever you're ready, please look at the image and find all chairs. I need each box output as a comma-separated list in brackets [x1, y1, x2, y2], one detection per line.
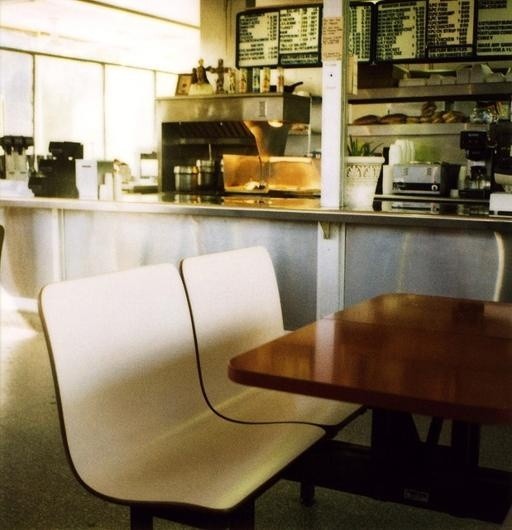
[177, 246, 368, 509]
[36, 260, 331, 528]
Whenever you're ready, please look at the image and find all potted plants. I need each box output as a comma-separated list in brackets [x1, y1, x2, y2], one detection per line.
[344, 135, 385, 211]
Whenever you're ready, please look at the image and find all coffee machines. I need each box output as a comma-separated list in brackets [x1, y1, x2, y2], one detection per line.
[458, 130, 498, 198]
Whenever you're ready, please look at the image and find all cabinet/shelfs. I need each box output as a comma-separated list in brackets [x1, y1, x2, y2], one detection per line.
[347, 81, 512, 133]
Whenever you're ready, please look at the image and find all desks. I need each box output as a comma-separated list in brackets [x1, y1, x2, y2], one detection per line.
[228, 291, 511, 526]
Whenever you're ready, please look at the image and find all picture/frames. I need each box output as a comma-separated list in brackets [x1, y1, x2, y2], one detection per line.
[176, 73, 192, 96]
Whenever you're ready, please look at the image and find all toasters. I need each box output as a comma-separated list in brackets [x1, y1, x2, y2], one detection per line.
[392, 163, 450, 195]
